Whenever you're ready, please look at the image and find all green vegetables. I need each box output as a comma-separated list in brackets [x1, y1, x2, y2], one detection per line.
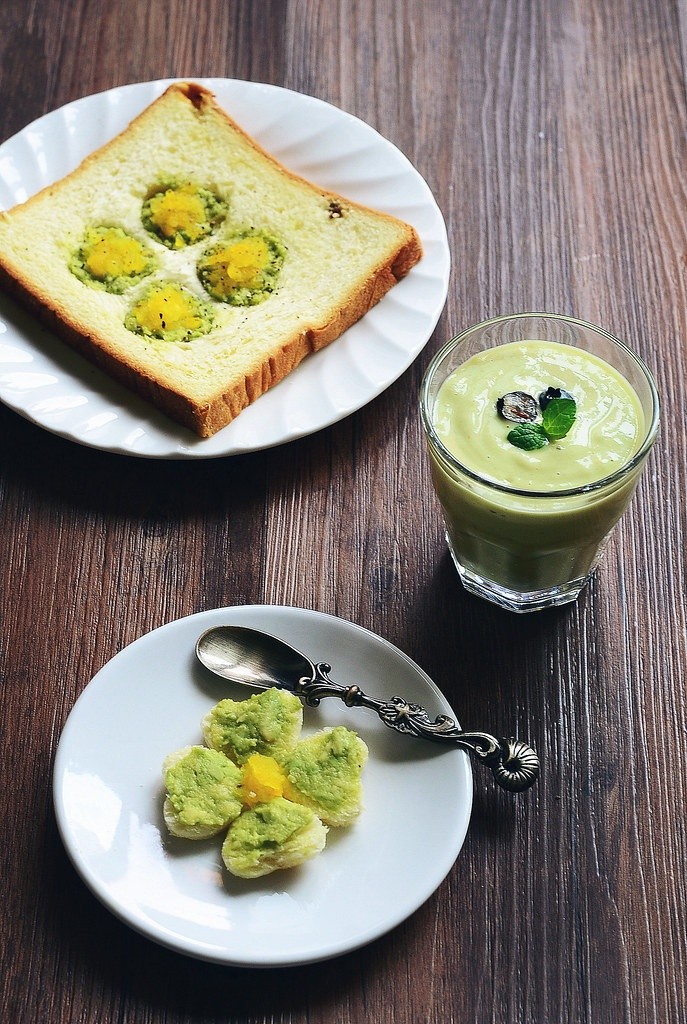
[507, 397, 576, 451]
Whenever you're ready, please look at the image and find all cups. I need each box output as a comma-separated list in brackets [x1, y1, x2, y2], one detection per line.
[417, 313, 661, 614]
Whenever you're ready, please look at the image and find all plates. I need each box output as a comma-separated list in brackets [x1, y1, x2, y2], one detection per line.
[0, 79, 449, 460]
[54, 603, 476, 958]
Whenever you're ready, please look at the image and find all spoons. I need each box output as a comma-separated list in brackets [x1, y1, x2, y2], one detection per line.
[192, 626, 540, 789]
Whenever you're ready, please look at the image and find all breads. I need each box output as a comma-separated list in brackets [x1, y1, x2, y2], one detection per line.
[0, 80, 423, 438]
